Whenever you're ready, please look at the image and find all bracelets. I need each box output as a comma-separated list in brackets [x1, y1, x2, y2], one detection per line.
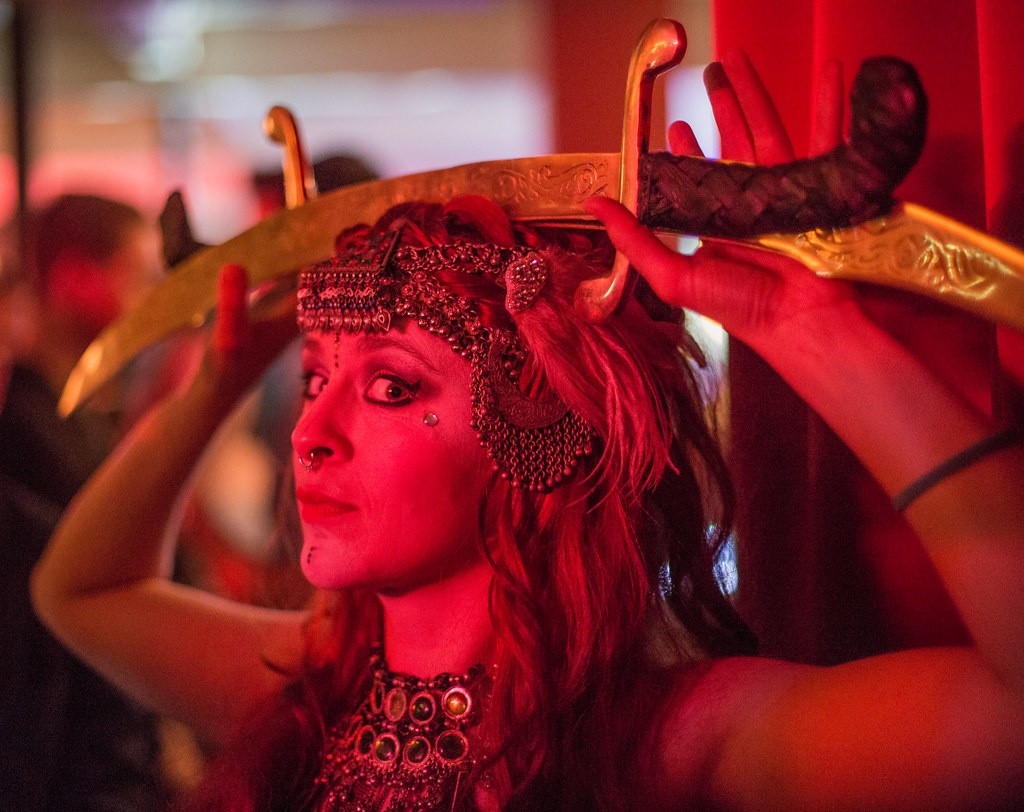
[887, 423, 1024, 520]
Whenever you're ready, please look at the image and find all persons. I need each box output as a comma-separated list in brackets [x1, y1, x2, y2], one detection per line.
[0, 135, 416, 812]
[28, 46, 1024, 812]
